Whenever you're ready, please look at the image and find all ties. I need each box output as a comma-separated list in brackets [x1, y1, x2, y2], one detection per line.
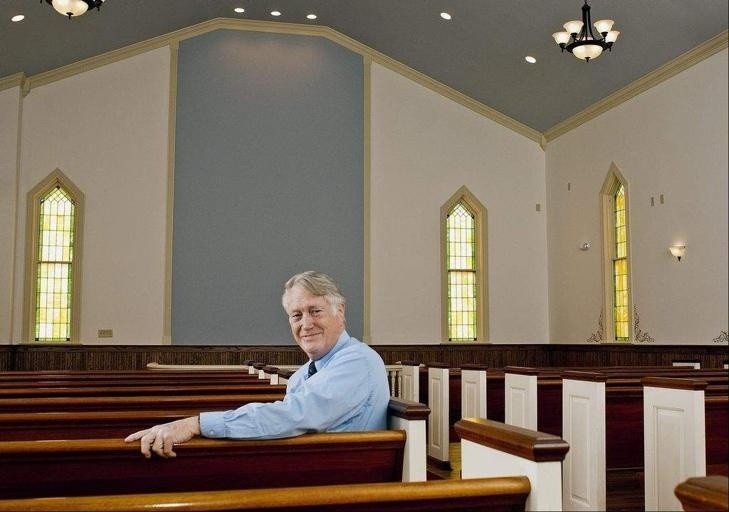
[307, 360, 316, 379]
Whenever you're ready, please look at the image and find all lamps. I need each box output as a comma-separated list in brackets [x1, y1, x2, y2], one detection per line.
[39, 0, 102, 21]
[551, 0, 621, 62]
[669, 245, 686, 262]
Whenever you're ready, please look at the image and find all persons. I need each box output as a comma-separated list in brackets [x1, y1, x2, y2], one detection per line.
[124, 269, 392, 459]
